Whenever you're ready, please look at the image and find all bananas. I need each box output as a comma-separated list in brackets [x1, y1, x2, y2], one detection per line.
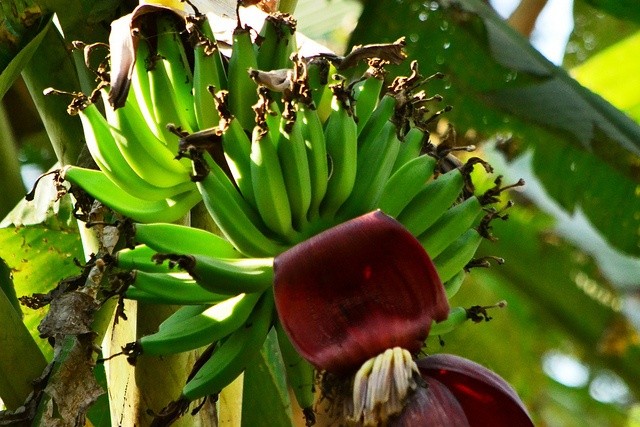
[42, 0, 384, 222]
[115, 56, 525, 422]
